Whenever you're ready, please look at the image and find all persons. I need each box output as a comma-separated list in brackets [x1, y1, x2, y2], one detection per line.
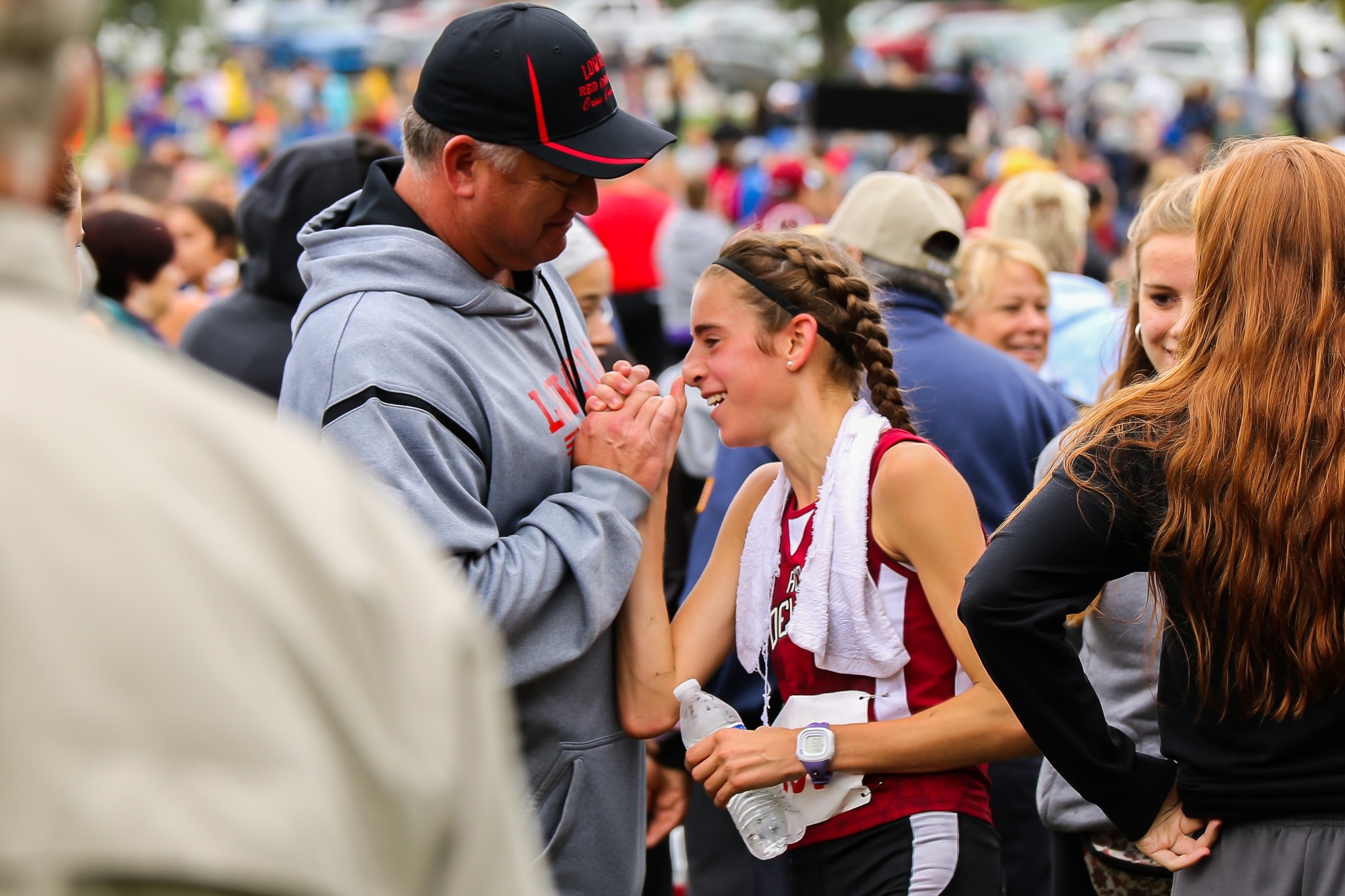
[0, 1, 1345, 896]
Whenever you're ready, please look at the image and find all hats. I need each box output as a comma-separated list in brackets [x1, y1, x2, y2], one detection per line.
[413, 3, 677, 180]
[799, 171, 967, 281]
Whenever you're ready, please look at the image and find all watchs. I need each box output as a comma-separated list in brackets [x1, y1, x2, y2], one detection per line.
[795, 721, 836, 786]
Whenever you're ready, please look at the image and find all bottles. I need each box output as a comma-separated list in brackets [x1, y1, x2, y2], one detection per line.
[672, 678, 807, 860]
